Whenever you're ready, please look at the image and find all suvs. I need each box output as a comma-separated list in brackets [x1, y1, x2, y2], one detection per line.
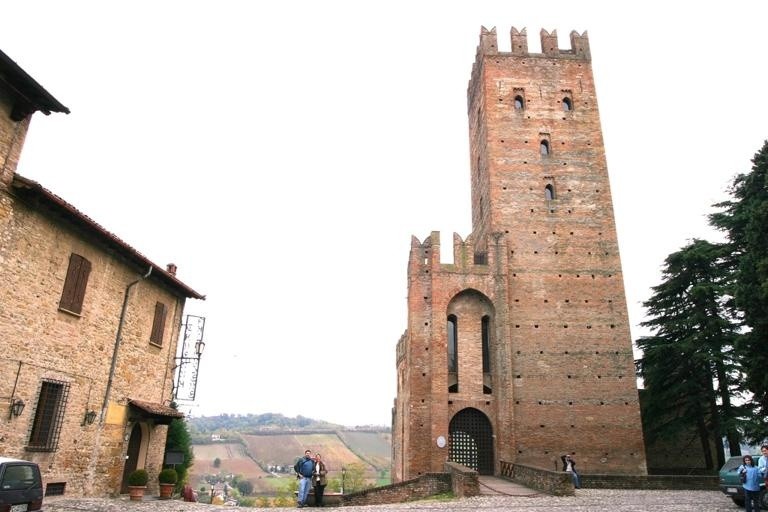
[718, 455, 768, 509]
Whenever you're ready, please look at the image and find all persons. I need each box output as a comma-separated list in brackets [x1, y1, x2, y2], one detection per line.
[736, 455, 766, 512]
[758, 445, 768, 491]
[561, 455, 580, 489]
[311, 454, 328, 507]
[294, 450, 315, 508]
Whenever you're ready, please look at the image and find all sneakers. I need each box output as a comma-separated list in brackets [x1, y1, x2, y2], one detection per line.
[575, 486, 580, 489]
[297, 503, 309, 508]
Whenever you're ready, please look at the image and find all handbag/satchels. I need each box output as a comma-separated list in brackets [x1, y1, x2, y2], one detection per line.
[740, 473, 746, 483]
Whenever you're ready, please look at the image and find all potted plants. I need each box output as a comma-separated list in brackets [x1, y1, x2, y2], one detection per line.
[157, 467, 179, 499]
[127, 469, 149, 501]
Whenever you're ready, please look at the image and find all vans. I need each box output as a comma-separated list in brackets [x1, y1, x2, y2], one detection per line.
[0, 456, 44, 512]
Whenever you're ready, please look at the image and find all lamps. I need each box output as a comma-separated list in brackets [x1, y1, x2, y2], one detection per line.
[80, 408, 97, 427]
[9, 398, 25, 420]
